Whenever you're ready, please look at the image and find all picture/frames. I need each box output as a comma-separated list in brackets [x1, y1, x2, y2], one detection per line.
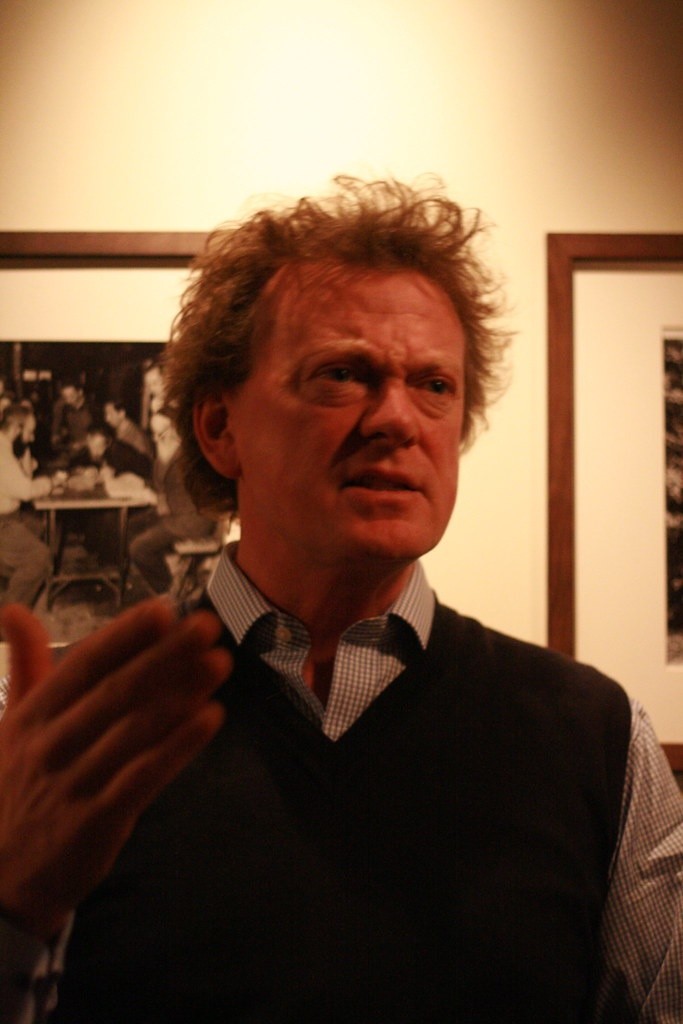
[0, 230, 244, 704]
[546, 229, 682, 779]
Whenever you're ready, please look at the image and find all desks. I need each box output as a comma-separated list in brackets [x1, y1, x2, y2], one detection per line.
[31, 497, 156, 615]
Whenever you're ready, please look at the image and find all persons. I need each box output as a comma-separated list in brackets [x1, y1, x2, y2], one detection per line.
[0, 171, 683, 1024]
[0, 351, 216, 614]
